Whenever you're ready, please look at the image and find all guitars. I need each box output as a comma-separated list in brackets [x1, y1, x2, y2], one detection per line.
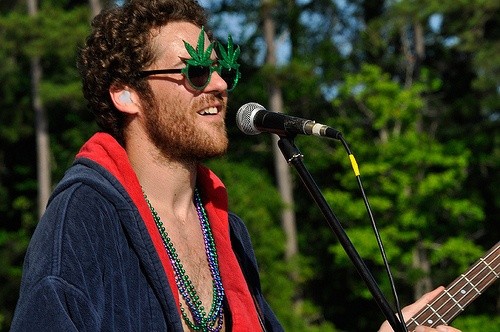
[394, 241, 500, 332]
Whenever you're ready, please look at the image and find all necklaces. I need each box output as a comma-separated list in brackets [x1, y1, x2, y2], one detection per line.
[95, 146, 231, 332]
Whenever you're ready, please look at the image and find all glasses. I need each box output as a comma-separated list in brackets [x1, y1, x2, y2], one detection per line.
[118, 25, 241, 92]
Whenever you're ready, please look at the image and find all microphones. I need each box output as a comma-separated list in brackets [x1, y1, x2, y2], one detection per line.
[235, 101, 342, 140]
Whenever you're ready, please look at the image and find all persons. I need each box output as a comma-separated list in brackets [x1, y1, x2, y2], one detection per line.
[11, 0, 462, 332]
